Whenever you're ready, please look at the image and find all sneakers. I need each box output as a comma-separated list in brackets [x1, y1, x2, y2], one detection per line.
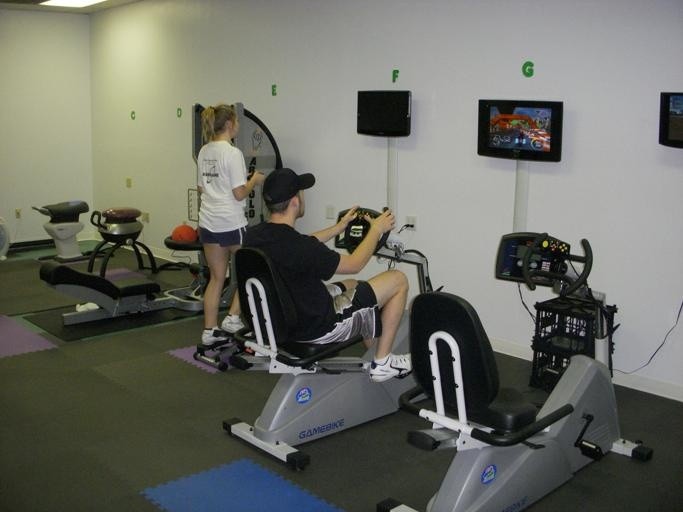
[219, 312, 247, 334]
[366, 352, 413, 385]
[198, 324, 233, 348]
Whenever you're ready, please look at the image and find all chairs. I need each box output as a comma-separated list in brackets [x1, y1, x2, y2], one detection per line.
[29, 253, 161, 317]
[231, 246, 351, 356]
[407, 289, 538, 435]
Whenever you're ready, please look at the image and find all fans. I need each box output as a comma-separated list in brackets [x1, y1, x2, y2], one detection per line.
[0, 216, 10, 261]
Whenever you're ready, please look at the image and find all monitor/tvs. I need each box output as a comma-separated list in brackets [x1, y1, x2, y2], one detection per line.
[659, 92, 683, 148]
[478, 99, 563, 162]
[357, 90, 411, 138]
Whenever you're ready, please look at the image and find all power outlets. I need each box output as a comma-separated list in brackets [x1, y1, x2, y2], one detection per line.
[15, 208, 20, 219]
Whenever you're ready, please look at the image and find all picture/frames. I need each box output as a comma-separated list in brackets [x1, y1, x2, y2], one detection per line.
[187, 188, 199, 222]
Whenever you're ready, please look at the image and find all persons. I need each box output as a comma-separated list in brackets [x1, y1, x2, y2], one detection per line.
[242, 167, 414, 383]
[197, 104, 266, 346]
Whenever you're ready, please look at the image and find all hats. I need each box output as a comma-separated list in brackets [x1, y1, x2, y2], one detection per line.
[260, 165, 317, 207]
